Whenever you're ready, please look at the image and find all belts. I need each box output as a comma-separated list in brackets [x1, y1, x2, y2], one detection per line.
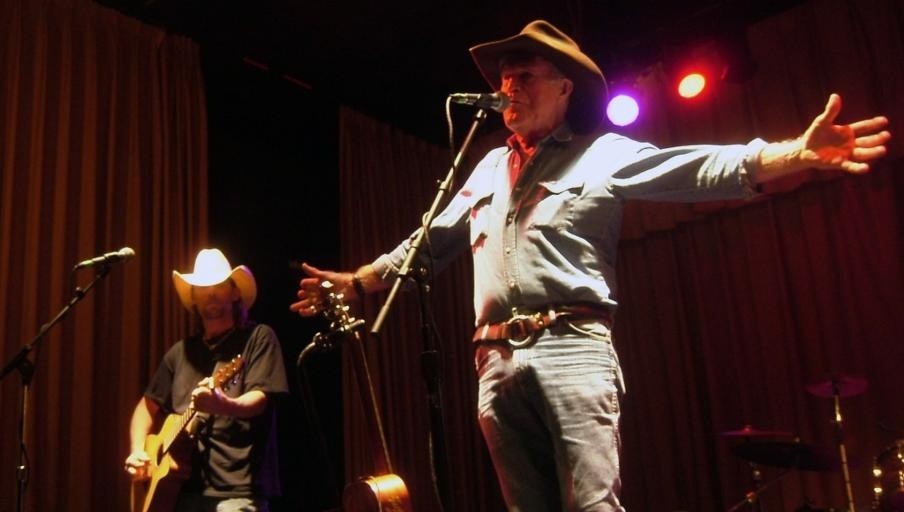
[471, 305, 611, 346]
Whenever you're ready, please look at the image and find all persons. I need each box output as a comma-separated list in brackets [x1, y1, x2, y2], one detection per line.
[288, 20, 891, 512]
[124, 277, 289, 512]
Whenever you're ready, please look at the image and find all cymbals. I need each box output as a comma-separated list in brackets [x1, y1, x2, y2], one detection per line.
[712, 430, 795, 436]
[731, 439, 842, 471]
[802, 370, 866, 399]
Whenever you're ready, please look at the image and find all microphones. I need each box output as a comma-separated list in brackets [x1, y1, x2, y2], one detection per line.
[452, 88, 511, 112]
[73, 245, 139, 267]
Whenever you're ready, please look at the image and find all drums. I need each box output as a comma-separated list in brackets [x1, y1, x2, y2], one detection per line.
[868, 440, 904, 512]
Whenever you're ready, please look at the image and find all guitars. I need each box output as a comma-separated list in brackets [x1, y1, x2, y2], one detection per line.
[131, 354, 247, 510]
[317, 281, 410, 510]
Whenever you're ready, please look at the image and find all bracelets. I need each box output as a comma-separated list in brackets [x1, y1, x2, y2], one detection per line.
[352, 271, 367, 299]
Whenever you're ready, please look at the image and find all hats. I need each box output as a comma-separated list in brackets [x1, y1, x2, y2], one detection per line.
[172, 248, 257, 310]
[469, 21, 608, 135]
